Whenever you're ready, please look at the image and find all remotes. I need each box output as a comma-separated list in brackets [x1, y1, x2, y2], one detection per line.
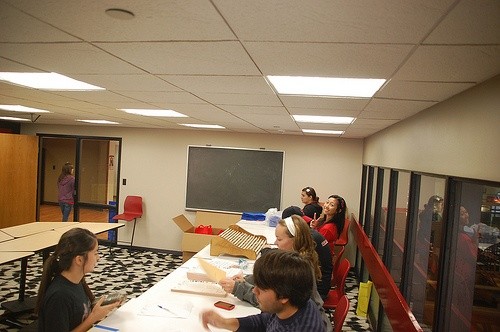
[91, 293, 123, 306]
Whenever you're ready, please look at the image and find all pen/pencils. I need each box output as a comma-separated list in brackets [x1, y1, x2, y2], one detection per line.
[157, 304, 171, 313]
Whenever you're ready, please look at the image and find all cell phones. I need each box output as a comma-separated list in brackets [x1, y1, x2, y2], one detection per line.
[214, 301, 235, 310]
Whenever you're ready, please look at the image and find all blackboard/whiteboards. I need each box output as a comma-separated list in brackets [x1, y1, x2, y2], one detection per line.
[184, 144, 286, 215]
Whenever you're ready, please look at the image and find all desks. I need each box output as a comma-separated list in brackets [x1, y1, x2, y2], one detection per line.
[0, 222, 125, 312]
[85, 219, 278, 332]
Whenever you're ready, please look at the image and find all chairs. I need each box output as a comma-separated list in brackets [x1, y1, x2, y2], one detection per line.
[323, 218, 351, 332]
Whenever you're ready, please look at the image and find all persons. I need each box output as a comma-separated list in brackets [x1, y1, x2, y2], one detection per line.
[57, 162, 75, 222]
[35, 228, 122, 332]
[201, 187, 347, 332]
[417, 196, 481, 287]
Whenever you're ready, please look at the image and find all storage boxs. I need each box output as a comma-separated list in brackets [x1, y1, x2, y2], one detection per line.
[171, 211, 242, 264]
[379, 207, 406, 229]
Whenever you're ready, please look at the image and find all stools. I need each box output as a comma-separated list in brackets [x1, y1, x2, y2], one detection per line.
[109, 195, 143, 257]
